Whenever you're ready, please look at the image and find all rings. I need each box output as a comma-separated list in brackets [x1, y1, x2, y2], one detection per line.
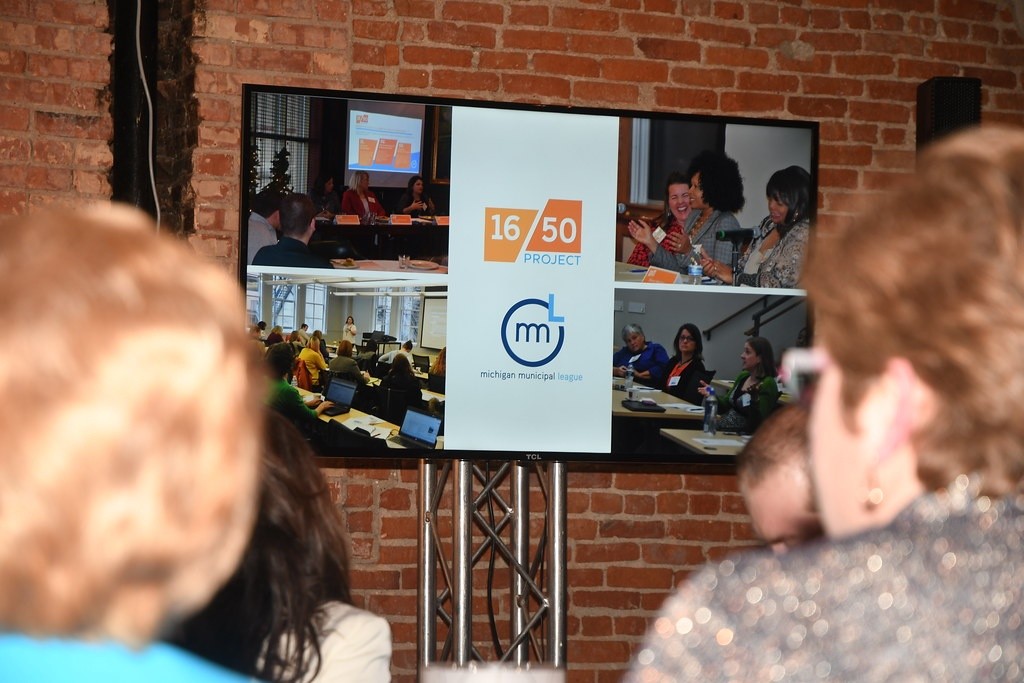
[715, 267, 717, 270]
[678, 243, 682, 248]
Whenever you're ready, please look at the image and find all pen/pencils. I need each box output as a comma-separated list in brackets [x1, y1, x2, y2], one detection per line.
[632, 269, 647, 272]
[690, 409, 703, 411]
[640, 388, 655, 390]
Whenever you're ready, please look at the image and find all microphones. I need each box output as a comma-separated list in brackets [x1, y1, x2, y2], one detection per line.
[716, 227, 761, 240]
[617, 202, 626, 213]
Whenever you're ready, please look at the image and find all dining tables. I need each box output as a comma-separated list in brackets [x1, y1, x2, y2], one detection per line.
[334, 259, 448, 274]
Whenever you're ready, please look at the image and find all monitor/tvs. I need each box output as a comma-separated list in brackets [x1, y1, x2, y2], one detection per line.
[238, 82, 820, 476]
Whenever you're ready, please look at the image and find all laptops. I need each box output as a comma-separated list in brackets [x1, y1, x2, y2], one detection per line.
[307, 377, 358, 416]
[386, 405, 442, 449]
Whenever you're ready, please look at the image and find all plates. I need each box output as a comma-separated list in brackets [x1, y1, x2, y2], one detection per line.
[409, 260, 440, 269]
[333, 263, 359, 270]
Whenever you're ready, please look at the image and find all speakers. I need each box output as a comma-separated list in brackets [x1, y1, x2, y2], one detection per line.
[916, 76, 982, 158]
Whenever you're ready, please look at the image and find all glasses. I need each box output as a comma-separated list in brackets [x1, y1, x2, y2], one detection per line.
[680, 335, 695, 341]
[780, 347, 833, 402]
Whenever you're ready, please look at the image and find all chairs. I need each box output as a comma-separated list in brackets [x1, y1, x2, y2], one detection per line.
[372, 360, 391, 378]
[354, 358, 372, 373]
[412, 353, 430, 373]
[428, 373, 445, 395]
[324, 369, 352, 396]
[373, 382, 408, 425]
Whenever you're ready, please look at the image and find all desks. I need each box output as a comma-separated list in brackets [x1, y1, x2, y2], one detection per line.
[295, 343, 445, 448]
[615, 258, 689, 284]
[611, 381, 793, 454]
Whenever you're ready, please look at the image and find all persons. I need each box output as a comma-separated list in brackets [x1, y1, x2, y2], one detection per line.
[248, 172, 436, 267]
[165, 411, 394, 683]
[627, 150, 811, 290]
[1, 198, 268, 683]
[628, 128, 1024, 683]
[249, 316, 445, 437]
[613, 325, 782, 432]
[737, 402, 824, 555]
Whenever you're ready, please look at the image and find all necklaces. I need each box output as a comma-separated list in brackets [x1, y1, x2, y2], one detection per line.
[691, 211, 713, 234]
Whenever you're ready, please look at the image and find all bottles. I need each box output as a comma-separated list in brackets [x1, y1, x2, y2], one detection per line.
[702, 387, 718, 437]
[688, 248, 702, 286]
[624, 363, 634, 390]
[333, 341, 336, 347]
[291, 376, 298, 389]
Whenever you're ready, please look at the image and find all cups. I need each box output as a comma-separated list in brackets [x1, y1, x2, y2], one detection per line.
[626, 387, 640, 401]
[416, 367, 420, 372]
[398, 255, 410, 270]
[312, 393, 321, 400]
